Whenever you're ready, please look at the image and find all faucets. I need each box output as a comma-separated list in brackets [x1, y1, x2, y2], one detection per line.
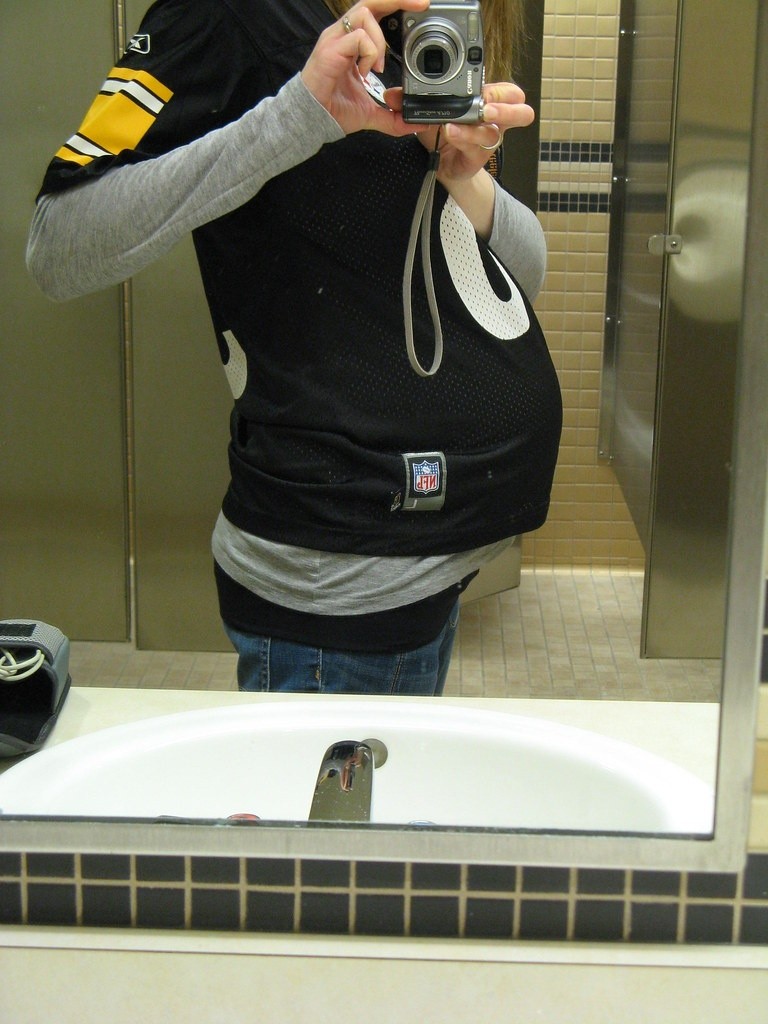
[309, 735, 391, 819]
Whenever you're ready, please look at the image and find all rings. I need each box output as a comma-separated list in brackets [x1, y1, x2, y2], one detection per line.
[478, 124, 503, 149]
[342, 17, 353, 33]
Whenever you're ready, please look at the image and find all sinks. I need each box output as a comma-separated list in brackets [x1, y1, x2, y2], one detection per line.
[0, 707, 676, 834]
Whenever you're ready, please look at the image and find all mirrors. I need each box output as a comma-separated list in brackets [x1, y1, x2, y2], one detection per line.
[0, 0, 768, 874]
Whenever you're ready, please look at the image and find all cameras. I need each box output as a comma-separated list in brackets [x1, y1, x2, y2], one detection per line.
[402, 0, 486, 124]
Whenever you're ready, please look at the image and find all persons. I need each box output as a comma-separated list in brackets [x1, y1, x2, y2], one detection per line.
[26, 0, 563, 697]
